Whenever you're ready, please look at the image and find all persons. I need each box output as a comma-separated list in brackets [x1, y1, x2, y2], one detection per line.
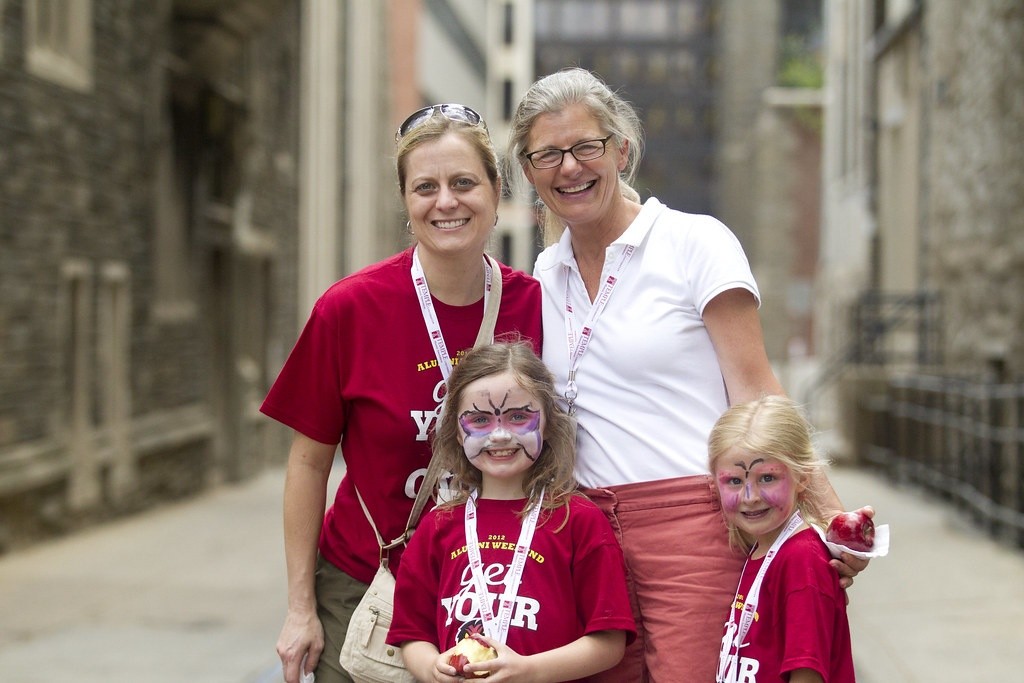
[708, 396, 860, 683]
[511, 65, 877, 682]
[384, 340, 636, 683]
[259, 101, 546, 683]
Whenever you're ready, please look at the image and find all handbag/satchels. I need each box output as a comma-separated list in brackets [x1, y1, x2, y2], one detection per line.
[339, 558, 419, 683]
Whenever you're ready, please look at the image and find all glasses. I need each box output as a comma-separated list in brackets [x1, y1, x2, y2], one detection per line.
[395, 104, 491, 149]
[522, 133, 614, 169]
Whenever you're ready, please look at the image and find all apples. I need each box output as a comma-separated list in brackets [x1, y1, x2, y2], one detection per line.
[448, 636, 497, 679]
[825, 511, 874, 553]
